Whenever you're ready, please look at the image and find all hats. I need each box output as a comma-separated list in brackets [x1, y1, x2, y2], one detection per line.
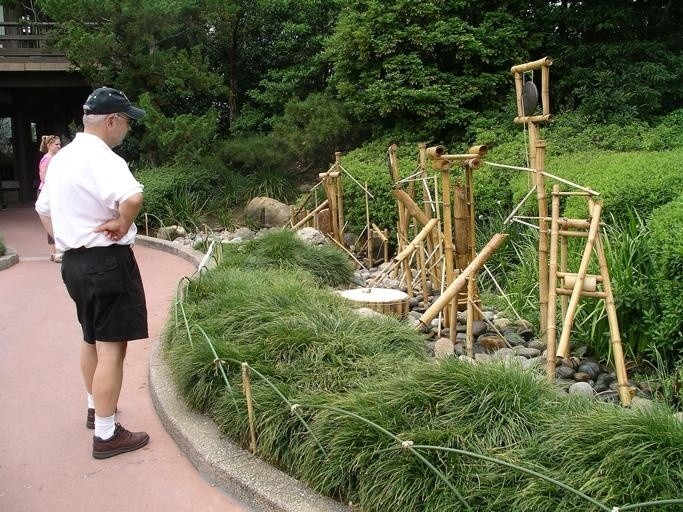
[84, 87, 146, 120]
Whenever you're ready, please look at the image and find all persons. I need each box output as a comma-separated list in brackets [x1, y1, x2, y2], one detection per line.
[35, 134, 64, 263]
[33, 86, 150, 458]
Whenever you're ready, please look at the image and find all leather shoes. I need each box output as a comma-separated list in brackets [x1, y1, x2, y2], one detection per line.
[87, 405, 149, 459]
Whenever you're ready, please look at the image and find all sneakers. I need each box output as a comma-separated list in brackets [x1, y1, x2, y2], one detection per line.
[50, 252, 63, 263]
